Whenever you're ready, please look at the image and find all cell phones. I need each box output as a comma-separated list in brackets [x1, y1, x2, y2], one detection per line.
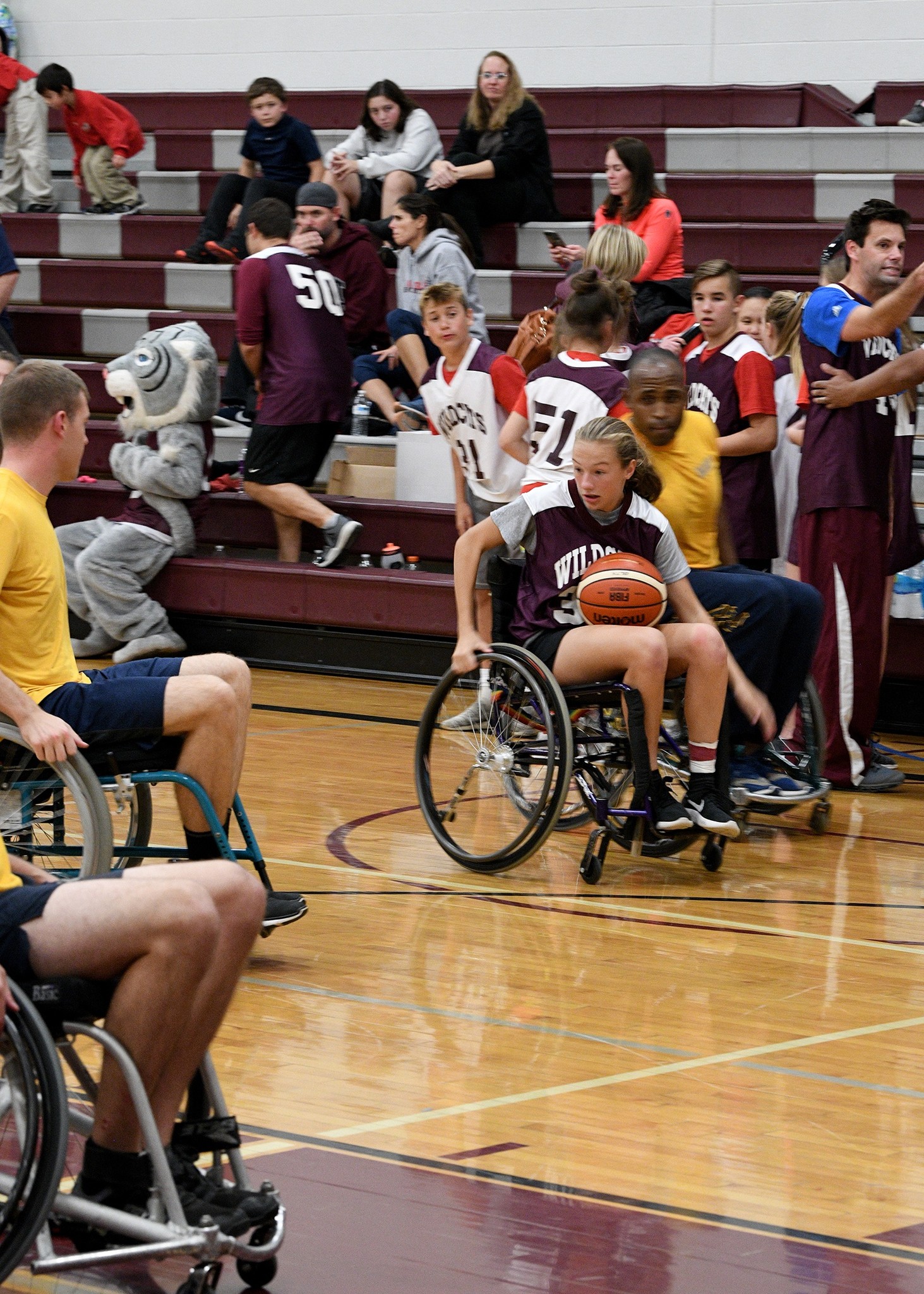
[543, 230, 565, 248]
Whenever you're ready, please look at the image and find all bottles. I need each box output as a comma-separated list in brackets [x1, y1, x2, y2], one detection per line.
[380, 543, 404, 570]
[358, 554, 374, 567]
[237, 449, 248, 492]
[351, 390, 370, 436]
[404, 556, 421, 571]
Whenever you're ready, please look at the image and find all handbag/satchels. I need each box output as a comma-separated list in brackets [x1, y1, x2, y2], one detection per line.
[506, 297, 560, 376]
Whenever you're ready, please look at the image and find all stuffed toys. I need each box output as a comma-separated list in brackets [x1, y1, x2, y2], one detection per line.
[55, 320, 221, 665]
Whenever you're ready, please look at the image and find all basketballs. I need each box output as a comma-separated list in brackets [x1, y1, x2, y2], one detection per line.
[577, 552, 668, 627]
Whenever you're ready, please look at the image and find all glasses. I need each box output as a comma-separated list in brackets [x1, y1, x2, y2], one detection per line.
[478, 72, 509, 79]
[818, 237, 843, 269]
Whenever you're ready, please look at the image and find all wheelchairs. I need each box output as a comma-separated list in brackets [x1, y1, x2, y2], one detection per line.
[411, 553, 835, 885]
[1, 709, 281, 942]
[0, 871, 289, 1293]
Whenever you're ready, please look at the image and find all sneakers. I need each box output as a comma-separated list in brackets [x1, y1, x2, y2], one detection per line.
[260, 888, 308, 928]
[204, 229, 251, 265]
[173, 235, 224, 263]
[651, 775, 693, 830]
[681, 783, 741, 838]
[727, 764, 783, 797]
[760, 763, 815, 796]
[316, 514, 364, 568]
[70, 1148, 281, 1253]
[210, 403, 257, 430]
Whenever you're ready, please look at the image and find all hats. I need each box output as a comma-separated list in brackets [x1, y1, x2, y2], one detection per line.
[295, 181, 338, 208]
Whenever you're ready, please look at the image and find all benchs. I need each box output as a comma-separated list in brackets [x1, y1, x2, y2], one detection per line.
[0, 82, 924, 744]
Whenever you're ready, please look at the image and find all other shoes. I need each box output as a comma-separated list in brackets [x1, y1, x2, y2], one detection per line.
[439, 699, 496, 730]
[81, 193, 149, 215]
[833, 762, 905, 791]
[510, 706, 546, 736]
[30, 204, 46, 213]
[391, 409, 430, 434]
[869, 747, 899, 769]
[392, 394, 430, 421]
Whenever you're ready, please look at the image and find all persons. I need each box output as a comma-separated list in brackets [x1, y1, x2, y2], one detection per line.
[0, 27, 924, 934]
[0, 835, 283, 1247]
